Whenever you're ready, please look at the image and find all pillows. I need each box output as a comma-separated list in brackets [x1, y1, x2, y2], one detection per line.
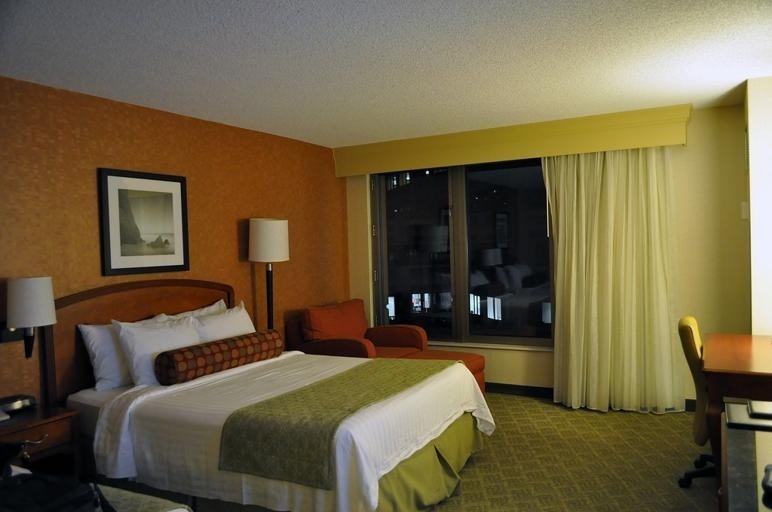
[108, 313, 203, 386]
[152, 327, 287, 388]
[165, 297, 229, 323]
[194, 297, 258, 342]
[76, 311, 170, 393]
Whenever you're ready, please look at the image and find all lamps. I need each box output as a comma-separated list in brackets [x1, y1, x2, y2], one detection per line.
[5, 275, 58, 360]
[247, 216, 291, 332]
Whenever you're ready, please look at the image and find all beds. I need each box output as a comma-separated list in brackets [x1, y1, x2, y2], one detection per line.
[38, 277, 498, 512]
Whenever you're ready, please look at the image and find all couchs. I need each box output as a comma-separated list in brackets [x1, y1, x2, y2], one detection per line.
[298, 296, 488, 397]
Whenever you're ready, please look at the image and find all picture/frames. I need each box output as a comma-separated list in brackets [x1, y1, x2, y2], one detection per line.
[94, 165, 191, 278]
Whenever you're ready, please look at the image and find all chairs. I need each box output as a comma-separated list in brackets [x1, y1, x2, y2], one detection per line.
[676, 314, 747, 501]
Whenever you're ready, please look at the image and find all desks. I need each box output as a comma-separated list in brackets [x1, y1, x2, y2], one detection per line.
[699, 333, 772, 512]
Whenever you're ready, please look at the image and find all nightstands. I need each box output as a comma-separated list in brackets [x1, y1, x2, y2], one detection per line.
[0, 398, 81, 465]
